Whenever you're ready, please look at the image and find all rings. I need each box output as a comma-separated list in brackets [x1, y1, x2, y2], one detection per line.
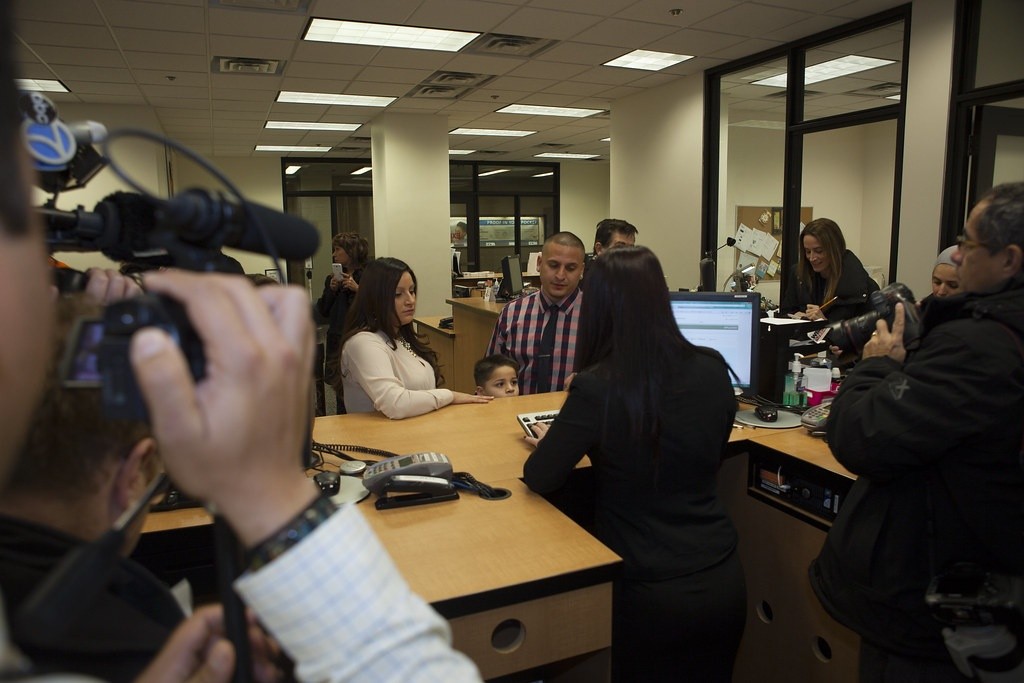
[871, 332, 877, 336]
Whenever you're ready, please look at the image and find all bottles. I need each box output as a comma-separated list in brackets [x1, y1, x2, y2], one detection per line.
[832, 368, 841, 391]
[810, 351, 832, 369]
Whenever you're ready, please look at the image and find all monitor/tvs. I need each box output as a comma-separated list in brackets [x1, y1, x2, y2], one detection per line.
[494, 254, 524, 302]
[667, 290, 761, 398]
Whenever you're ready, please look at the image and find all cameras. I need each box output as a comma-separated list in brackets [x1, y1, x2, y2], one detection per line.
[823, 282, 924, 361]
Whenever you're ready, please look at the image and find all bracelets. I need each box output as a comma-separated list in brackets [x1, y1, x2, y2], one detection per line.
[236, 494, 337, 569]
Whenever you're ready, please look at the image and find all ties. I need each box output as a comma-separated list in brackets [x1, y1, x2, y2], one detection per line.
[537, 303, 560, 393]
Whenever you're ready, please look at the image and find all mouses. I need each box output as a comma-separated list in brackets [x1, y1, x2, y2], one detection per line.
[755, 406, 778, 422]
[311, 470, 340, 495]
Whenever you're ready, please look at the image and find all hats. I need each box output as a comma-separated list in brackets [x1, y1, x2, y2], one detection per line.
[932, 244, 958, 274]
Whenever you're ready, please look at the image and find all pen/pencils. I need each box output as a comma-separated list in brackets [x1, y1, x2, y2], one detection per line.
[820, 296, 838, 309]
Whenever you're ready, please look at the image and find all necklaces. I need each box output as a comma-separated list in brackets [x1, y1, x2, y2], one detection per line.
[397, 337, 417, 357]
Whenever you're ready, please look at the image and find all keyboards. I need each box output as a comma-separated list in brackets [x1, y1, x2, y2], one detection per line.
[150, 487, 201, 514]
[517, 409, 561, 439]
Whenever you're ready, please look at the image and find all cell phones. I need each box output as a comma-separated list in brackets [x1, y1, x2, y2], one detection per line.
[332, 264, 343, 281]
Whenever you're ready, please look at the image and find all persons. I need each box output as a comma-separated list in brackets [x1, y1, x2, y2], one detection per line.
[811, 183, 1024, 683]
[483, 232, 585, 395]
[920, 244, 963, 307]
[315, 233, 371, 413]
[334, 258, 495, 420]
[522, 243, 747, 683]
[452, 221, 467, 243]
[781, 218, 880, 323]
[579, 219, 638, 288]
[0, 0, 479, 683]
[473, 354, 521, 398]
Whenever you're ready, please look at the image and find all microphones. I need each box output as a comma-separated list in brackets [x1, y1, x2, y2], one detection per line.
[166, 186, 322, 261]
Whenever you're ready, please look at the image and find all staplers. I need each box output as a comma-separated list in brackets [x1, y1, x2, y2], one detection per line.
[375, 475, 460, 510]
